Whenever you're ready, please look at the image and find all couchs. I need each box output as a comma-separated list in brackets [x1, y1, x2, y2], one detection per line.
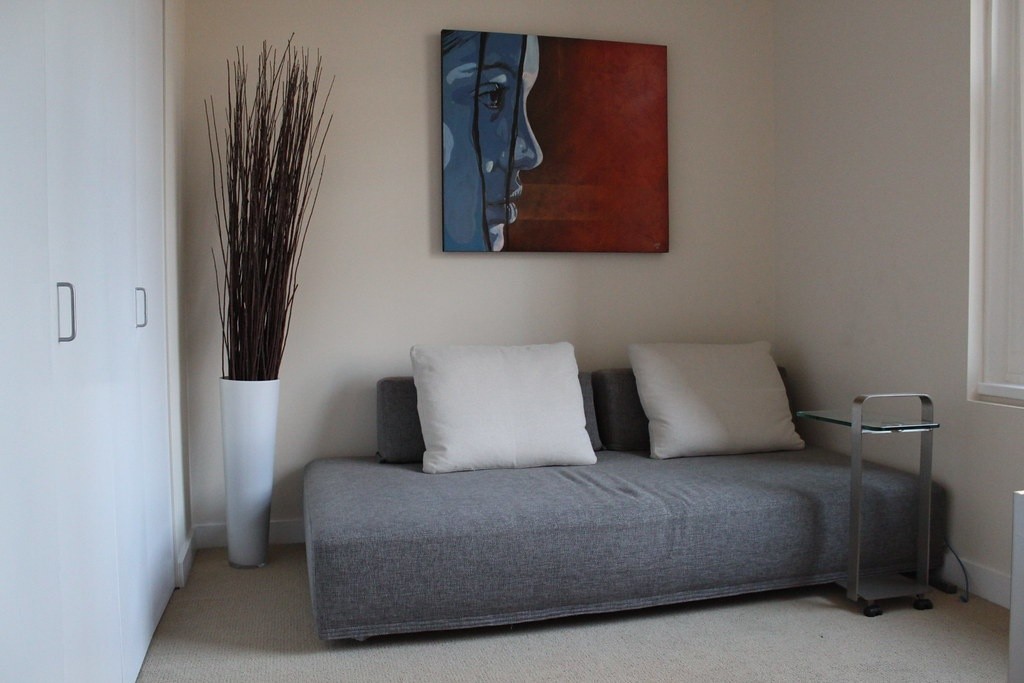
[301, 341, 946, 644]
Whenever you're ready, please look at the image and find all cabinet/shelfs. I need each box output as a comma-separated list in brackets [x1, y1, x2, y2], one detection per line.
[0, 0, 178, 683]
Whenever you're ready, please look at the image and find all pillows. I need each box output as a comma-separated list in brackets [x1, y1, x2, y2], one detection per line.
[628, 341, 805, 460]
[410, 342, 597, 474]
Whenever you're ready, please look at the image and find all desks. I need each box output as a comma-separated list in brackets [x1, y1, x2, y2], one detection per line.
[798, 393, 939, 618]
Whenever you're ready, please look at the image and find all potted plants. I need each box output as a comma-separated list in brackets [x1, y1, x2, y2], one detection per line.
[202, 28, 336, 568]
